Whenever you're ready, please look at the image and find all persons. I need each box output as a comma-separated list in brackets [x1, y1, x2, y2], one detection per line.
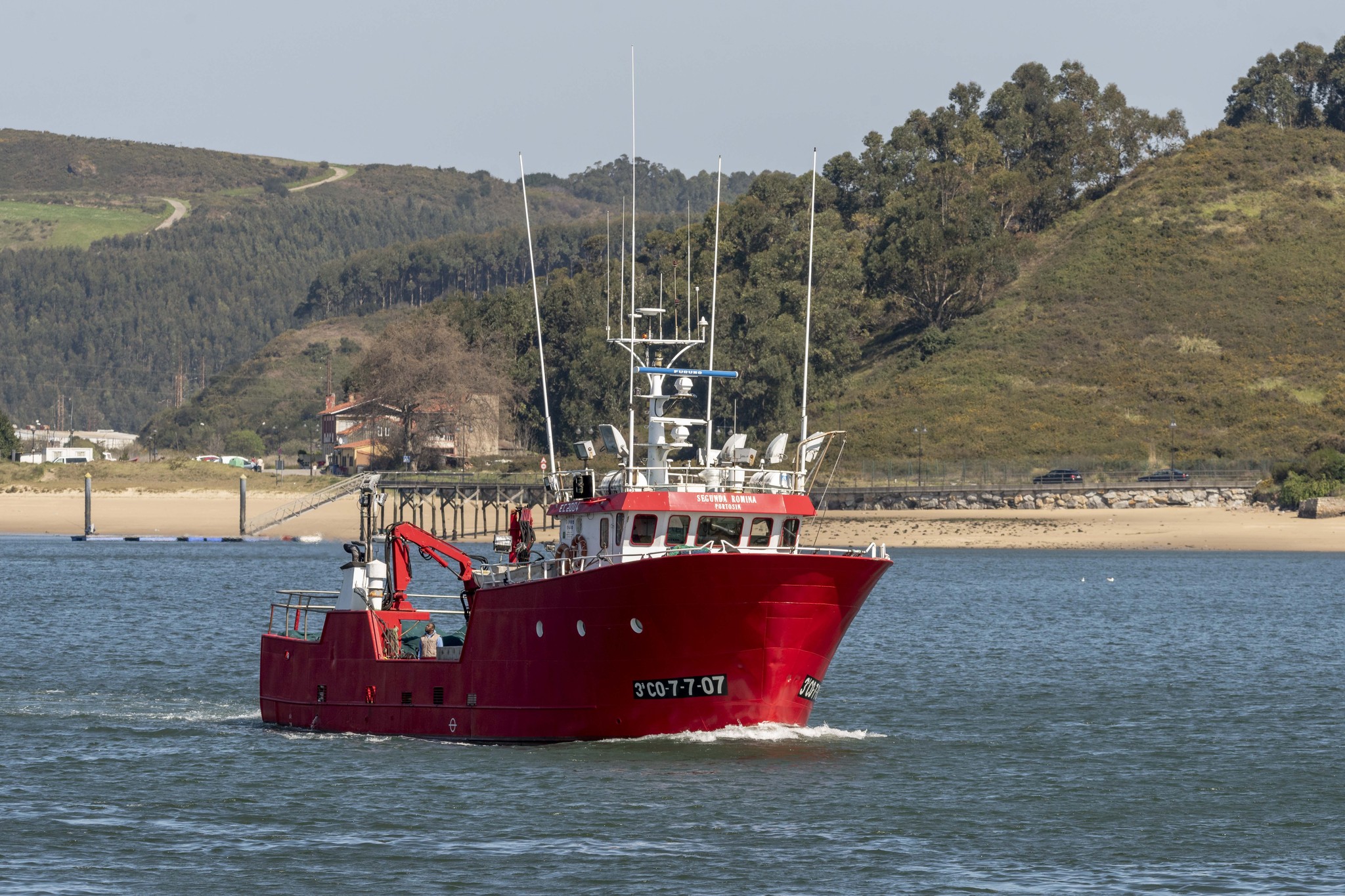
[252, 456, 255, 462]
[257, 457, 263, 472]
[418, 623, 443, 660]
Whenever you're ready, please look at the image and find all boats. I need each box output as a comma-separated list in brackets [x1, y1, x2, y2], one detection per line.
[258, 46, 894, 746]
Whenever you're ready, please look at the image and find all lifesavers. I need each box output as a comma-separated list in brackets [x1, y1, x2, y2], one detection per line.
[569, 533, 588, 569]
[554, 543, 571, 577]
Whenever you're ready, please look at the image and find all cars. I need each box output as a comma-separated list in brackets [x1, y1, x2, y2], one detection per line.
[1138, 469, 1192, 482]
[190, 455, 258, 472]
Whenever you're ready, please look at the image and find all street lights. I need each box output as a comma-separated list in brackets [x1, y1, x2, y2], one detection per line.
[303, 414, 320, 476]
[716, 418, 735, 450]
[31, 428, 37, 463]
[272, 418, 289, 462]
[912, 416, 928, 487]
[455, 416, 473, 484]
[1170, 419, 1176, 470]
[153, 429, 157, 462]
[148, 436, 152, 463]
[576, 418, 595, 470]
[68, 428, 75, 449]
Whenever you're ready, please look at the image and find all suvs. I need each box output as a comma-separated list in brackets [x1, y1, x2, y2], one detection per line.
[1032, 469, 1084, 484]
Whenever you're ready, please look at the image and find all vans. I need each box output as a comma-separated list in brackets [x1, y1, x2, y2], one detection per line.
[53, 457, 92, 465]
